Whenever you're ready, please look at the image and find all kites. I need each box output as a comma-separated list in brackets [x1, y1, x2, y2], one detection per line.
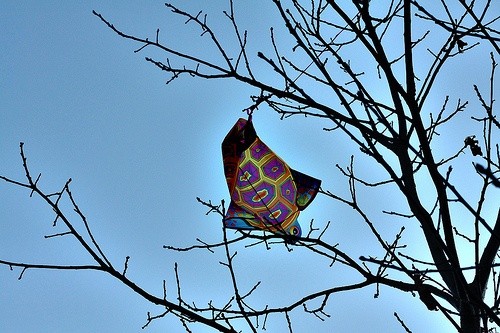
[222, 117, 323, 244]
